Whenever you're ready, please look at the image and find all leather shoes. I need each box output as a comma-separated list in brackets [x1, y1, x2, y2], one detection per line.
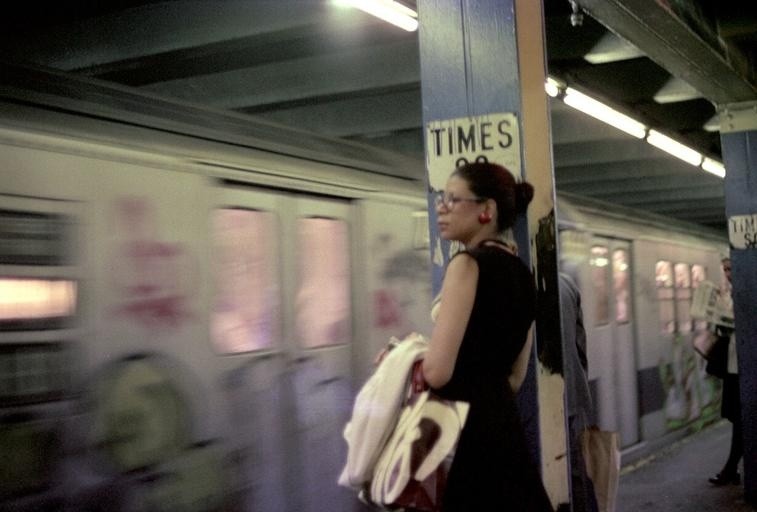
[706, 468, 741, 486]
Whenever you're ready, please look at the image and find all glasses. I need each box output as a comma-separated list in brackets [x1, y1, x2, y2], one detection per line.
[430, 191, 487, 212]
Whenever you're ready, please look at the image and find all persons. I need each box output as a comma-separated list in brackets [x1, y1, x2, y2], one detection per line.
[557, 268, 598, 511]
[690, 254, 744, 487]
[369, 161, 554, 511]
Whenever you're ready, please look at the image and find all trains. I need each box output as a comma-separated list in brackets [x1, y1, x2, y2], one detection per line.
[1, 62, 736, 512]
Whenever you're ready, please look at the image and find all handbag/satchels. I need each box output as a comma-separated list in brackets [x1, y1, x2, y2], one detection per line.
[580, 424, 626, 511]
[358, 355, 455, 511]
